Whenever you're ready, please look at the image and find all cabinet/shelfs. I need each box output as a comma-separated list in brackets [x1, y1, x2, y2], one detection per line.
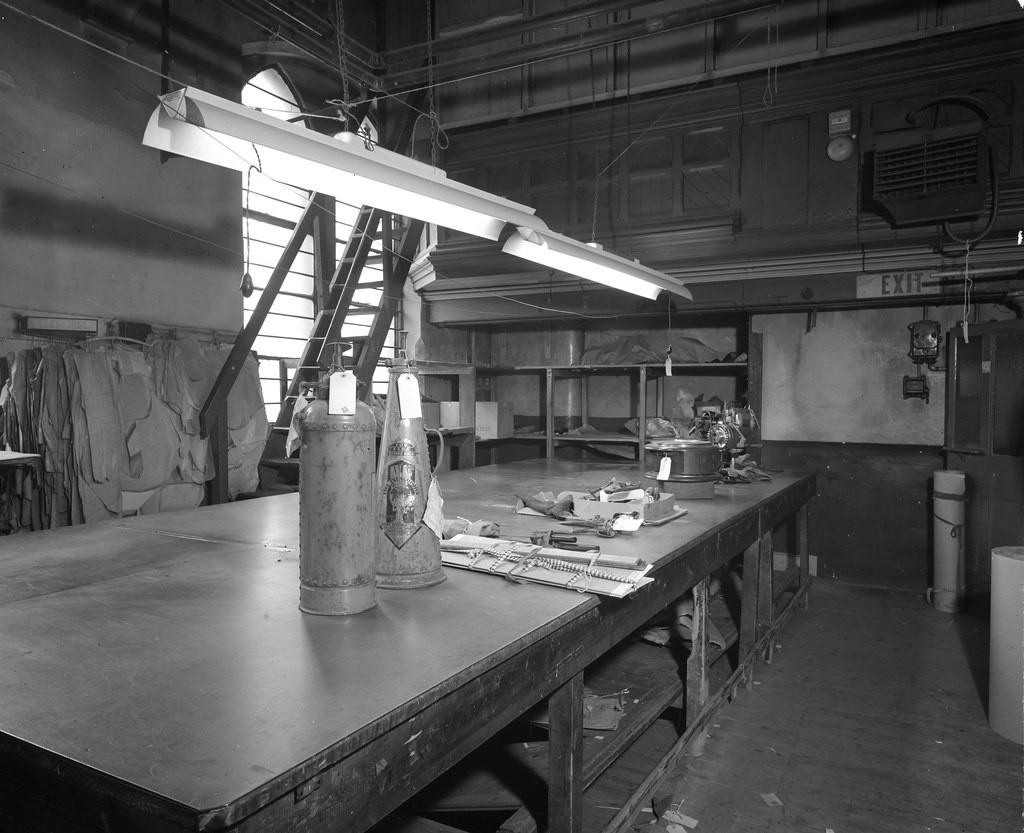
[412, 361, 478, 469]
[942, 319, 1023, 621]
[491, 362, 763, 475]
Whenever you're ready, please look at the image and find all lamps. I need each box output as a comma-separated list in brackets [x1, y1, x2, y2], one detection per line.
[498, 222, 694, 303]
[141, 87, 550, 244]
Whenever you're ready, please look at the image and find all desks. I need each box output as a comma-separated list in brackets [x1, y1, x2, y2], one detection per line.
[0, 458, 817, 832]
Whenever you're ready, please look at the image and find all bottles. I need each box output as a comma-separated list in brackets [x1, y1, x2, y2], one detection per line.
[376, 331, 446, 588]
[293, 342, 375, 616]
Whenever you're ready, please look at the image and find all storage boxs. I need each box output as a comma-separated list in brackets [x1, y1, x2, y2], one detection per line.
[440, 401, 514, 442]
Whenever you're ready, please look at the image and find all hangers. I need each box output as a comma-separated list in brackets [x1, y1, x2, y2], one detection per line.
[75, 324, 152, 351]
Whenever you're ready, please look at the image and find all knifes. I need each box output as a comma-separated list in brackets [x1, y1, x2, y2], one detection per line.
[491, 530, 599, 552]
